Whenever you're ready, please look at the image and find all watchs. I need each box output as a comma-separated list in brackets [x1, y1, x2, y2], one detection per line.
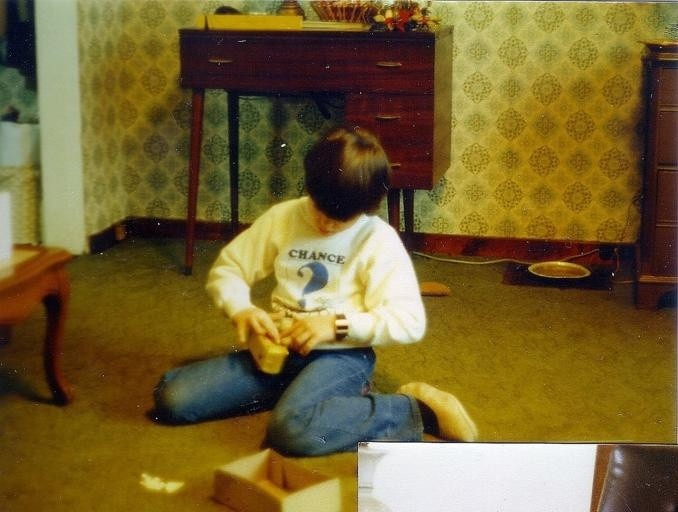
[335, 313, 347, 342]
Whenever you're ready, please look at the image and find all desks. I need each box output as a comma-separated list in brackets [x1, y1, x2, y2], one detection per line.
[0, 245, 75, 408]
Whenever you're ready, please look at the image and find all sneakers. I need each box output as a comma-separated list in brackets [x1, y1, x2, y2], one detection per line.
[398, 383, 477, 442]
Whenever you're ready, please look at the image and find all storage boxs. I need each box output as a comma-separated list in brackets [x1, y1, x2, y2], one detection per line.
[214, 448, 342, 511]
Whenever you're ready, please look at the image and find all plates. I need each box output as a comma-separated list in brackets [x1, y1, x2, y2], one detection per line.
[528, 260, 592, 278]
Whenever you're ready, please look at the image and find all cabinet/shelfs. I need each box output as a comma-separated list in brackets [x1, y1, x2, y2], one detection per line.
[640, 40, 678, 310]
[181, 27, 451, 275]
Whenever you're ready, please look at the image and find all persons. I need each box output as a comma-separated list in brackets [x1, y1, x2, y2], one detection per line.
[153, 130, 479, 460]
